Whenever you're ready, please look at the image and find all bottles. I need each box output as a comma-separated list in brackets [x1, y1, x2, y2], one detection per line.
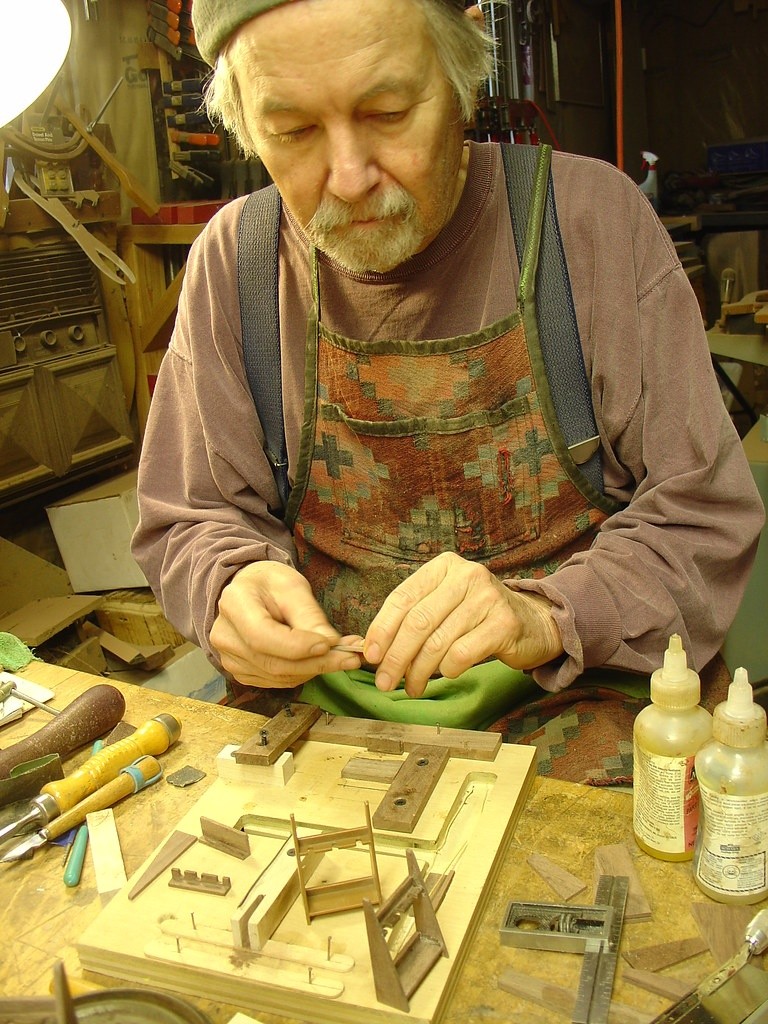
[691, 667, 768, 906]
[632, 632, 715, 863]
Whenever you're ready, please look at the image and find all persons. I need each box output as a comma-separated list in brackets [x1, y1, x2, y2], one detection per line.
[129, 0, 766, 794]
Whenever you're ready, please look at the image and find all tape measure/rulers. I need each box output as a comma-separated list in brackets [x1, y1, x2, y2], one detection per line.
[573, 874, 630, 1024]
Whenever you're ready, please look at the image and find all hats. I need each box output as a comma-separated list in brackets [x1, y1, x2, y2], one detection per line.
[191, 0, 475, 64]
